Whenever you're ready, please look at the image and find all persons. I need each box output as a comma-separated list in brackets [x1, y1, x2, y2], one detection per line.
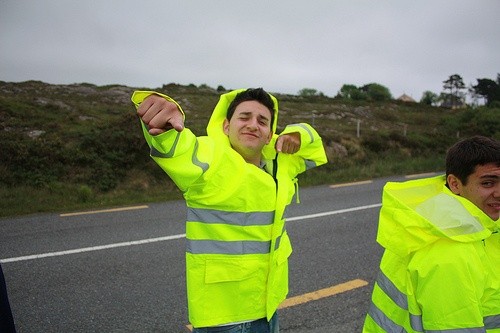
[137, 88, 327, 333]
[361, 137, 500, 333]
